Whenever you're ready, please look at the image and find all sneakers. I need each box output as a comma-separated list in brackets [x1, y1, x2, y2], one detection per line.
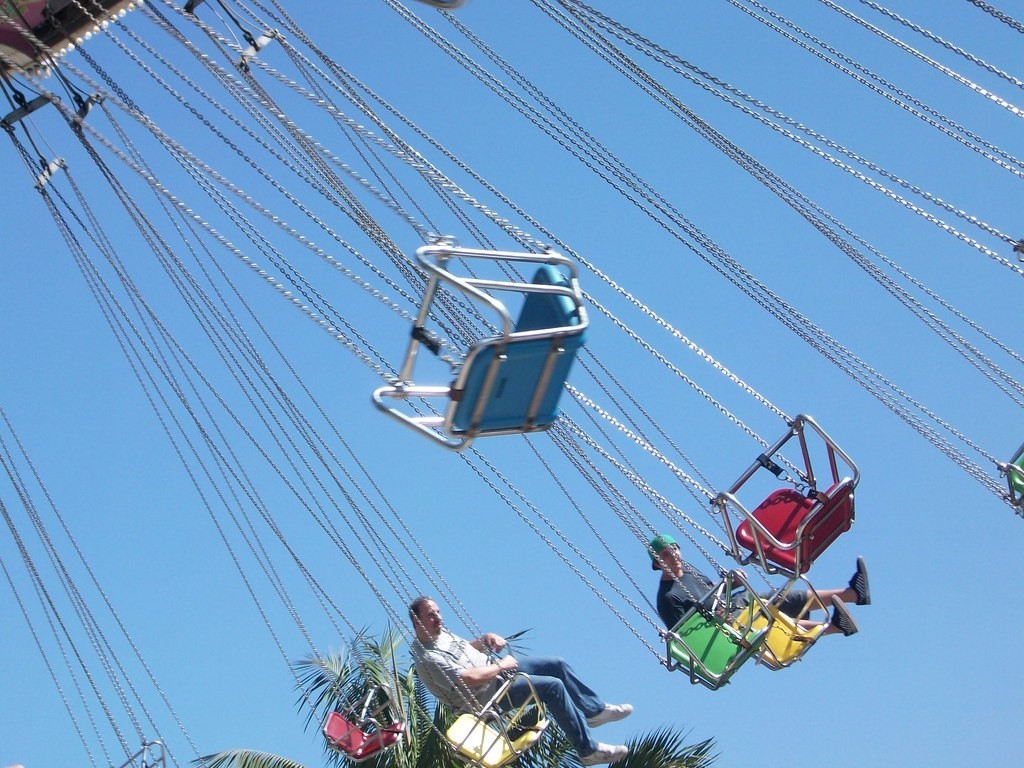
[579, 742, 628, 767]
[586, 703, 633, 728]
[831, 594, 858, 638]
[850, 556, 871, 605]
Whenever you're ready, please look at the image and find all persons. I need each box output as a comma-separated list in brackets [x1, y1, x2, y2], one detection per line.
[407, 597, 634, 765]
[647, 534, 872, 636]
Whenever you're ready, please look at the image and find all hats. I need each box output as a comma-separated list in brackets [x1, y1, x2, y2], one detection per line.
[649, 535, 679, 570]
